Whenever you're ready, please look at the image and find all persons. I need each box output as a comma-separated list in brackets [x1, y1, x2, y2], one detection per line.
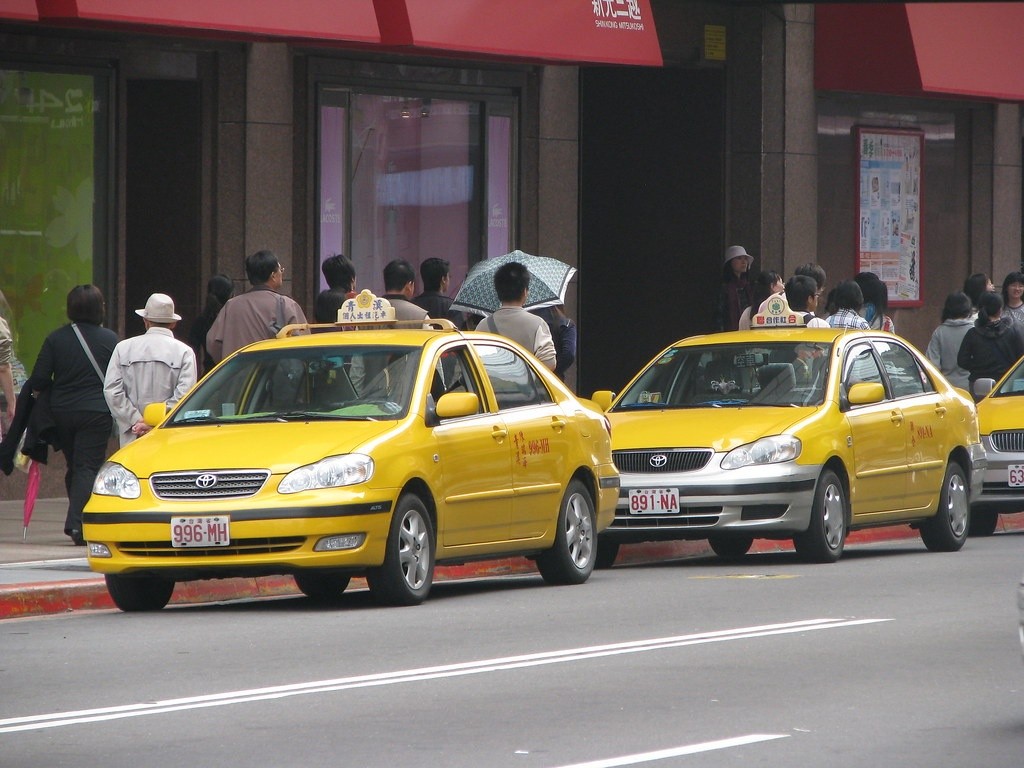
[29, 284, 118, 547]
[0, 317, 17, 443]
[189, 252, 310, 378]
[103, 293, 197, 449]
[313, 254, 467, 402]
[476, 264, 555, 376]
[711, 245, 895, 384]
[926, 271, 1024, 403]
[531, 306, 577, 384]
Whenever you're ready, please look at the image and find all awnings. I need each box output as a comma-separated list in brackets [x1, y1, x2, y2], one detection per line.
[0, 0, 664, 68]
[813, 3, 1024, 100]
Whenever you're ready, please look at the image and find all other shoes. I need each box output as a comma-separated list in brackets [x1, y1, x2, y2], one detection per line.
[70, 530, 87, 546]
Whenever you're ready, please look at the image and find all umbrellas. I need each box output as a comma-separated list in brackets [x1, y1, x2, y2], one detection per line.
[449, 250, 577, 319]
[23, 462, 42, 540]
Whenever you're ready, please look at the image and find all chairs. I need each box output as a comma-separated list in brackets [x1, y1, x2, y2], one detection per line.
[812, 356, 830, 387]
[271, 357, 308, 407]
[388, 351, 446, 401]
[750, 363, 797, 403]
[689, 360, 749, 400]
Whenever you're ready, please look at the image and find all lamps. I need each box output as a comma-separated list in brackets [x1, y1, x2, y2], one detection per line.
[400, 102, 410, 118]
[421, 97, 431, 117]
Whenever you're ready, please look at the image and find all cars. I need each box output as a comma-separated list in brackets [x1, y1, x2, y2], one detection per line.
[967, 354, 1024, 537]
[80, 329, 620, 611]
[591, 326, 985, 563]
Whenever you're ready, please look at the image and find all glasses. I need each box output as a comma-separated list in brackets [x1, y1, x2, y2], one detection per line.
[813, 292, 821, 298]
[279, 268, 285, 274]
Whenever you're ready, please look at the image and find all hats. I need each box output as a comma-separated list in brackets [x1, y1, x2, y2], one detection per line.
[135, 293, 182, 323]
[723, 245, 753, 270]
